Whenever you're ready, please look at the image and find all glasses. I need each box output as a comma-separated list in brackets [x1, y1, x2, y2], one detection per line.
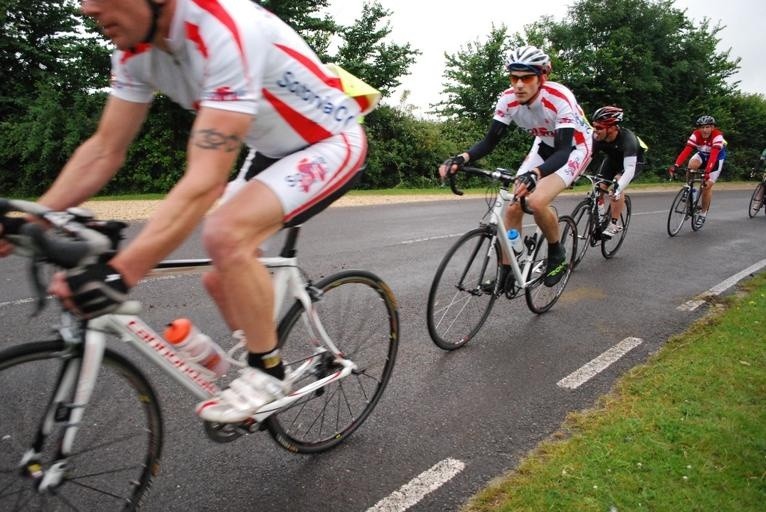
[592, 124, 613, 130]
[508, 71, 541, 85]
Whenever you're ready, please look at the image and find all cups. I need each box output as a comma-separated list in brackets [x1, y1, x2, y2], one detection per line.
[693, 189, 697, 202]
[508, 228, 524, 253]
[598, 199, 605, 216]
[165, 318, 229, 381]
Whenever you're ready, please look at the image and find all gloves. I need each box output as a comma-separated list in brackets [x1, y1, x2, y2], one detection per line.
[751, 167, 757, 176]
[609, 184, 622, 202]
[60, 261, 130, 320]
[668, 166, 677, 176]
[443, 154, 465, 178]
[701, 171, 710, 181]
[0, 209, 31, 246]
[514, 171, 538, 195]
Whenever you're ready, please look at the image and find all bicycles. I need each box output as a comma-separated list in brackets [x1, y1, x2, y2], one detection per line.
[668, 165, 709, 237]
[0, 197, 400, 511]
[427, 156, 578, 350]
[561, 174, 631, 267]
[749, 168, 766, 218]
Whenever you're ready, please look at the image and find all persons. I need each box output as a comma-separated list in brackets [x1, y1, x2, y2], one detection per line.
[669, 115, 728, 229]
[586, 105, 644, 239]
[753, 149, 766, 211]
[439, 45, 594, 294]
[1, 0, 369, 425]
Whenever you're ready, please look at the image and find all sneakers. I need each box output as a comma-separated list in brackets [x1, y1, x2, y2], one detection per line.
[693, 212, 706, 229]
[281, 354, 291, 378]
[481, 274, 516, 295]
[194, 359, 293, 425]
[544, 252, 569, 288]
[753, 200, 760, 210]
[690, 186, 698, 204]
[602, 222, 619, 238]
[596, 204, 606, 224]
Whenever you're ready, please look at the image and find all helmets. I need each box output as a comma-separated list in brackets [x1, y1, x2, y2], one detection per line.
[592, 105, 625, 129]
[504, 45, 553, 78]
[695, 115, 716, 128]
